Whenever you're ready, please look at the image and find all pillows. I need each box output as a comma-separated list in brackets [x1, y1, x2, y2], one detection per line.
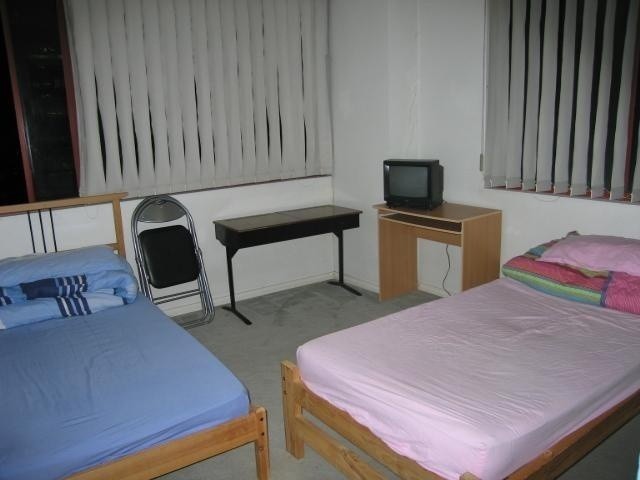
[535, 229, 640, 276]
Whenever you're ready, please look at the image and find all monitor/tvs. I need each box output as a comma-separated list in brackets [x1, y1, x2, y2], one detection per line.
[384, 159, 443, 210]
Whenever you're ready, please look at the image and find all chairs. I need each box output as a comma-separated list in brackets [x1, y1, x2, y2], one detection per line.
[129, 193, 215, 331]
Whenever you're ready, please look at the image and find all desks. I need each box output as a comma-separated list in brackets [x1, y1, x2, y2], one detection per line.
[371, 202, 502, 301]
[209, 205, 362, 326]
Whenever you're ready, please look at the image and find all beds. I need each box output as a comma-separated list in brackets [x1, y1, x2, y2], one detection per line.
[279, 230, 640, 480]
[0, 192, 271, 480]
